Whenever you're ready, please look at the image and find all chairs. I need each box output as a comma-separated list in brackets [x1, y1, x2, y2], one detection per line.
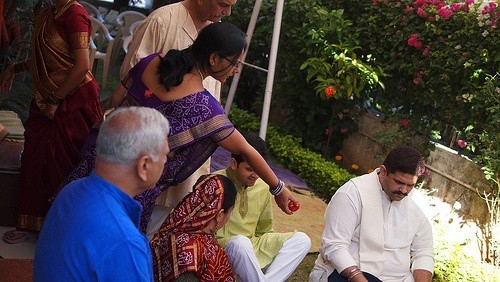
[78, 0, 146, 88]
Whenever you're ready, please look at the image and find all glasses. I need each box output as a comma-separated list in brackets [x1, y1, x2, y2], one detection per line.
[216, 50, 242, 68]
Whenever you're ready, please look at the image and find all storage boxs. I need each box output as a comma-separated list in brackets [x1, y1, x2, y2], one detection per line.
[0, 111, 26, 172]
